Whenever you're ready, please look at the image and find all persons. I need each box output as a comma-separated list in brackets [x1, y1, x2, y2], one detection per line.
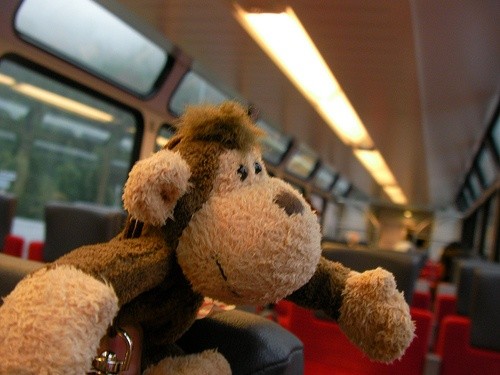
[345, 222, 429, 257]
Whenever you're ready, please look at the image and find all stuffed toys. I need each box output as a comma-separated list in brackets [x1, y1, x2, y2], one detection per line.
[0, 101, 418, 375]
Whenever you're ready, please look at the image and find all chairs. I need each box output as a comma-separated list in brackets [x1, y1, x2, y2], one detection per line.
[0, 190, 500, 375]
